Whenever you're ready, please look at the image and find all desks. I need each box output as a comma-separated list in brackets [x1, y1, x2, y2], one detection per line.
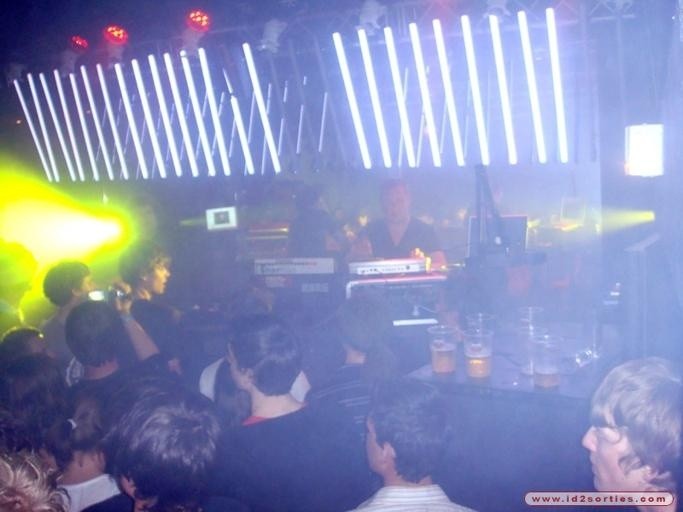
[404, 351, 600, 401]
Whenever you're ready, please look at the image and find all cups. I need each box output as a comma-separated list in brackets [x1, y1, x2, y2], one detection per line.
[465, 312, 495, 331]
[516, 304, 564, 390]
[464, 331, 492, 378]
[427, 324, 456, 374]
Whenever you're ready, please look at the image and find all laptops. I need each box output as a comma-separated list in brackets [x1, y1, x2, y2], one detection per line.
[466, 217, 527, 268]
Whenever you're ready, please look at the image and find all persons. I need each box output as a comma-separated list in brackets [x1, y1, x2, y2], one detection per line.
[0, 176, 683, 512]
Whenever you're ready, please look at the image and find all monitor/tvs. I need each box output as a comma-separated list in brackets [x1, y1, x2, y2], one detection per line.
[205, 205, 238, 231]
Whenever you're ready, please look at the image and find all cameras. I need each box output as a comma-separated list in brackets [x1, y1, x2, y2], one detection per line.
[87, 286, 129, 305]
